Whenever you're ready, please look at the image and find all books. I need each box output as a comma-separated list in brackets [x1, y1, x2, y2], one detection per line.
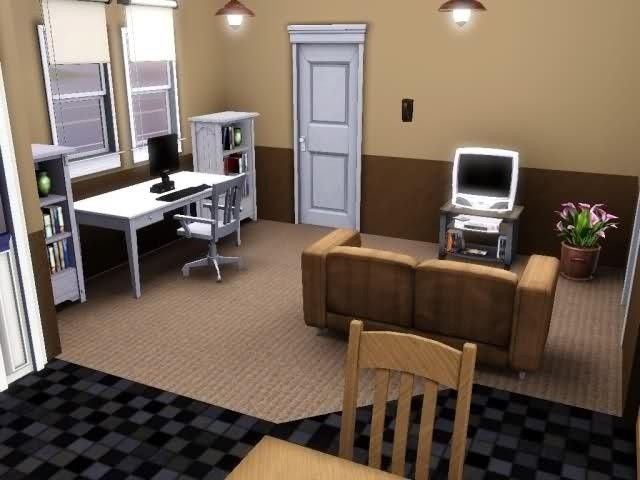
[42, 205, 70, 275]
